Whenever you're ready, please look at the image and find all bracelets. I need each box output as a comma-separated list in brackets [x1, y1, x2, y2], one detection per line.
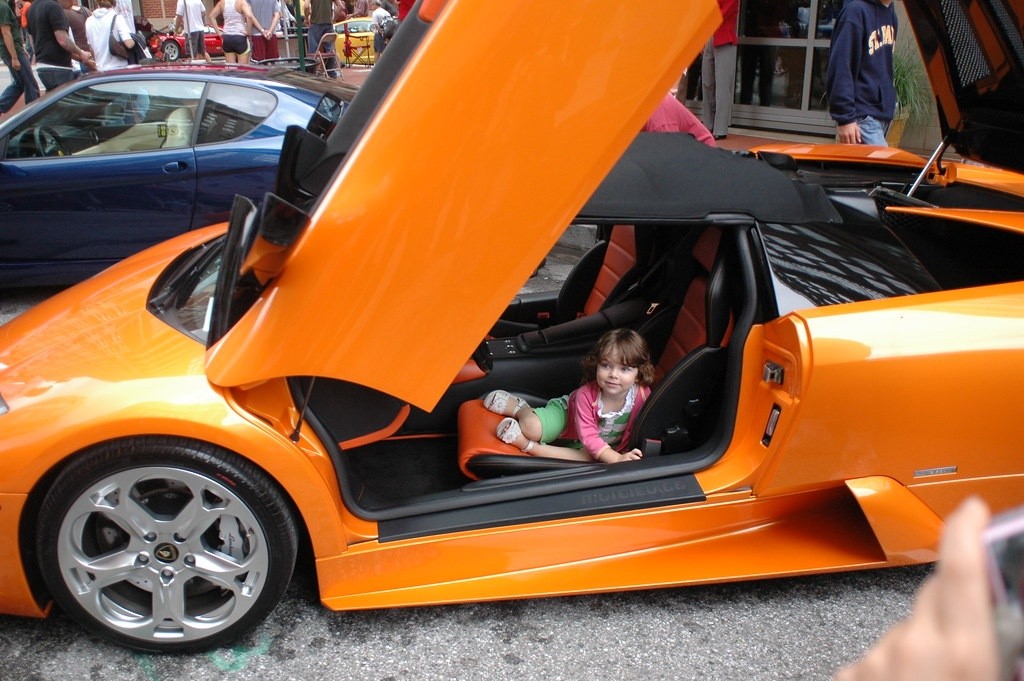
[261, 29, 265, 34]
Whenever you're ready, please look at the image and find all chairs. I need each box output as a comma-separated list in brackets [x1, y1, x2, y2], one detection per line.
[484, 225, 663, 342]
[342, 23, 372, 69]
[207, 104, 251, 143]
[456, 225, 759, 481]
[161, 107, 193, 148]
[305, 33, 344, 81]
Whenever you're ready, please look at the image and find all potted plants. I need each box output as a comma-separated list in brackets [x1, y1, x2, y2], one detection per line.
[814, 19, 935, 148]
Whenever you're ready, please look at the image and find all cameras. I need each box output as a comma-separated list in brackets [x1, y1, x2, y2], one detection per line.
[977, 507, 1024, 672]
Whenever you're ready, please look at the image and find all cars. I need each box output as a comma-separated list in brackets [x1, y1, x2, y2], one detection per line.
[1, 63, 365, 292]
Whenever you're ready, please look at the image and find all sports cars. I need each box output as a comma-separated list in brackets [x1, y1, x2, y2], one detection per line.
[150, 20, 225, 60]
[333, 16, 383, 65]
[0, 0, 1024, 656]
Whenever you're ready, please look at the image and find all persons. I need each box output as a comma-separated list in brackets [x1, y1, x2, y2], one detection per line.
[209, 0, 399, 81]
[0, 0, 146, 126]
[173, 0, 212, 64]
[701, 1, 741, 141]
[686, 0, 804, 106]
[484, 329, 655, 465]
[824, 0, 898, 148]
[829, 494, 1024, 680]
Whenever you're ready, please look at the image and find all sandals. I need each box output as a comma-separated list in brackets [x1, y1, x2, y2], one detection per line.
[496, 418, 534, 452]
[483, 390, 531, 417]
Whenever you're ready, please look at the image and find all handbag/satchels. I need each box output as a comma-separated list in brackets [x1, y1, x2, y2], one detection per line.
[109, 14, 128, 59]
